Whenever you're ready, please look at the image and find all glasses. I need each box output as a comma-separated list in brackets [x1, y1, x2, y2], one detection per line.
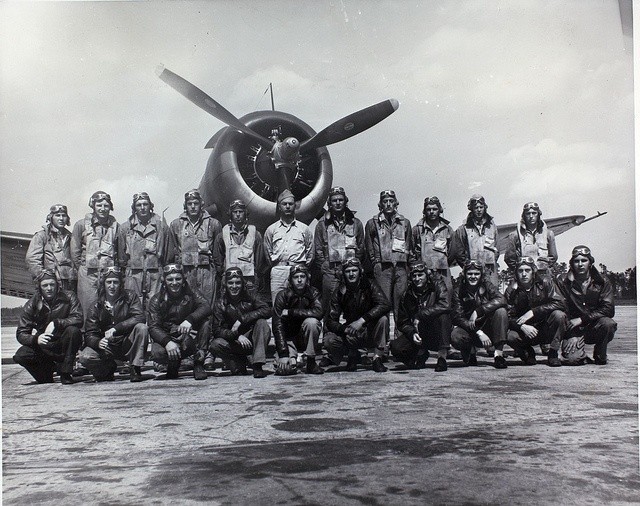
[103, 266, 121, 275]
[523, 203, 539, 209]
[380, 191, 395, 196]
[330, 187, 344, 193]
[342, 258, 359, 266]
[230, 200, 244, 207]
[409, 264, 424, 272]
[50, 206, 67, 212]
[572, 249, 590, 255]
[185, 192, 199, 198]
[93, 194, 110, 201]
[163, 264, 184, 273]
[516, 257, 534, 264]
[464, 260, 482, 266]
[225, 270, 242, 277]
[470, 198, 485, 205]
[133, 192, 149, 198]
[425, 196, 439, 202]
[37, 269, 53, 279]
[290, 264, 308, 272]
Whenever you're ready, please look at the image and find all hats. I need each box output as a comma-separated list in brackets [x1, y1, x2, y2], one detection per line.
[522, 202, 542, 219]
[288, 264, 311, 288]
[378, 195, 399, 211]
[34, 271, 62, 293]
[277, 189, 294, 204]
[327, 192, 349, 209]
[408, 260, 431, 281]
[227, 205, 250, 223]
[89, 191, 113, 211]
[569, 245, 594, 270]
[468, 194, 488, 210]
[422, 201, 443, 214]
[463, 259, 483, 278]
[131, 196, 154, 214]
[184, 192, 205, 213]
[342, 257, 364, 279]
[100, 272, 125, 288]
[223, 267, 247, 292]
[514, 256, 537, 281]
[46, 205, 70, 227]
[162, 263, 188, 290]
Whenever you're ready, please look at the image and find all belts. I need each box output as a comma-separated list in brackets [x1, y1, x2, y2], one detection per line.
[276, 261, 297, 265]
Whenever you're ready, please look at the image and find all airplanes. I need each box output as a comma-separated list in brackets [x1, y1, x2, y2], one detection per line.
[0, 55, 610, 303]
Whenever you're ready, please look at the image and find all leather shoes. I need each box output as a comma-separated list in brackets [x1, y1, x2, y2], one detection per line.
[593, 345, 607, 365]
[372, 358, 387, 372]
[448, 352, 463, 359]
[130, 365, 142, 382]
[106, 364, 117, 381]
[435, 357, 447, 371]
[494, 356, 507, 369]
[153, 363, 166, 372]
[489, 351, 507, 358]
[512, 348, 520, 356]
[527, 347, 537, 365]
[194, 363, 208, 379]
[346, 351, 359, 372]
[306, 364, 324, 374]
[541, 344, 551, 355]
[253, 365, 264, 378]
[204, 363, 215, 370]
[319, 356, 331, 366]
[72, 368, 89, 376]
[167, 359, 180, 379]
[119, 367, 130, 374]
[60, 373, 73, 384]
[468, 352, 477, 366]
[222, 360, 229, 370]
[548, 351, 561, 366]
[415, 351, 430, 369]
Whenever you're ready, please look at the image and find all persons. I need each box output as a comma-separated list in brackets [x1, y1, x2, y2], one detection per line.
[409, 195, 465, 361]
[79, 269, 149, 382]
[212, 199, 263, 371]
[504, 256, 569, 366]
[450, 259, 509, 368]
[169, 188, 224, 371]
[69, 190, 121, 376]
[209, 266, 273, 378]
[24, 203, 78, 376]
[263, 188, 316, 371]
[146, 262, 213, 380]
[322, 257, 391, 373]
[555, 245, 618, 365]
[389, 260, 451, 372]
[364, 187, 413, 361]
[503, 201, 559, 358]
[313, 185, 366, 368]
[271, 263, 327, 375]
[454, 193, 509, 358]
[11, 267, 85, 385]
[117, 191, 175, 375]
